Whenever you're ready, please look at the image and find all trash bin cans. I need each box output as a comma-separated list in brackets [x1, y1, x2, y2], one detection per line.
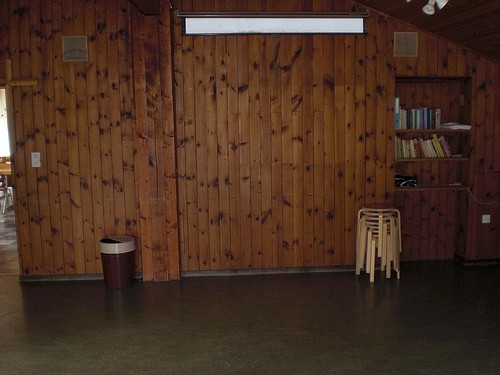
[99, 235, 137, 289]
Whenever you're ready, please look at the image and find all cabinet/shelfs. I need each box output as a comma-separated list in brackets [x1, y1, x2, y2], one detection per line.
[394, 127, 472, 192]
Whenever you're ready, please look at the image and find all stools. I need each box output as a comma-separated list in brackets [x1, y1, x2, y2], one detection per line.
[353, 204, 404, 283]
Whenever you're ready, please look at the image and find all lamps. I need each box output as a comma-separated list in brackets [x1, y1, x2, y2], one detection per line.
[422, 0, 449, 15]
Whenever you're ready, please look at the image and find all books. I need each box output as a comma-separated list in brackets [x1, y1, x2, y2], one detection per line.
[395, 134, 464, 158]
[394, 95, 472, 131]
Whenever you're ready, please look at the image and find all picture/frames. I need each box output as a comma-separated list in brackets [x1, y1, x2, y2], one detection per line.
[62, 34, 88, 63]
[393, 32, 418, 58]
